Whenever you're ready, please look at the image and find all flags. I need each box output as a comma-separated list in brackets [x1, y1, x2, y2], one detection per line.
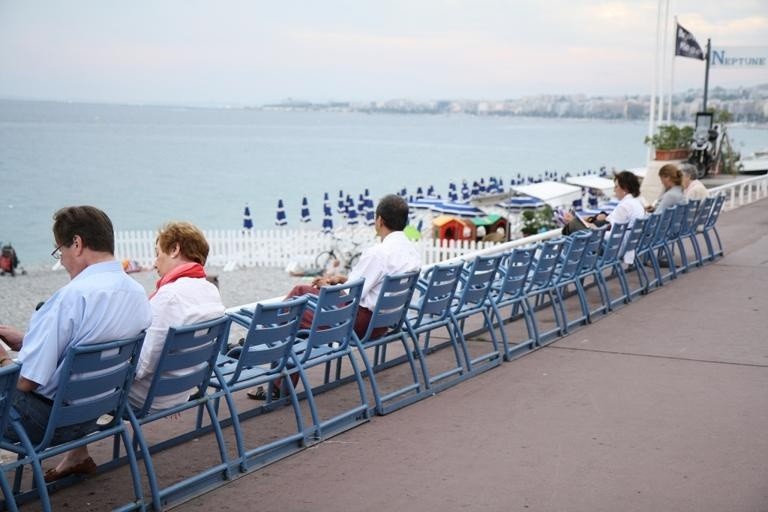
[676, 23, 704, 61]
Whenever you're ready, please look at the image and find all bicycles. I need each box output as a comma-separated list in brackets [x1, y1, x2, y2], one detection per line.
[316, 236, 363, 272]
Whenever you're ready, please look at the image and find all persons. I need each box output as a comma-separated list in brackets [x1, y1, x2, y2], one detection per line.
[43, 222, 224, 481]
[246, 195, 422, 400]
[707, 125, 719, 156]
[0, 206, 151, 449]
[652, 164, 684, 215]
[680, 164, 707, 203]
[604, 170, 646, 265]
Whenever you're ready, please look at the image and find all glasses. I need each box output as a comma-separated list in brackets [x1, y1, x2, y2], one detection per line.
[52, 233, 77, 259]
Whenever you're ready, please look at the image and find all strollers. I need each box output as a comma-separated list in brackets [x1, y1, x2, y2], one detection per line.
[0, 242, 28, 276]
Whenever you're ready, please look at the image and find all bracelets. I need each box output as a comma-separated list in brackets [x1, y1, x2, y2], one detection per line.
[0, 354, 11, 366]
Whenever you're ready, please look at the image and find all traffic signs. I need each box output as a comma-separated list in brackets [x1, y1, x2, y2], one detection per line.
[711, 47, 768, 68]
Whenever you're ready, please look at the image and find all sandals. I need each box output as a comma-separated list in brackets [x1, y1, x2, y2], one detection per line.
[44, 456, 97, 482]
[247, 381, 289, 400]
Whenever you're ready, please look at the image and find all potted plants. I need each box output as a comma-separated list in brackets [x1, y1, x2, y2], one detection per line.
[643, 124, 697, 160]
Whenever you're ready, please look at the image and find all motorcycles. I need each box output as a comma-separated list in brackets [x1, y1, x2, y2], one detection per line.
[686, 125, 719, 179]
